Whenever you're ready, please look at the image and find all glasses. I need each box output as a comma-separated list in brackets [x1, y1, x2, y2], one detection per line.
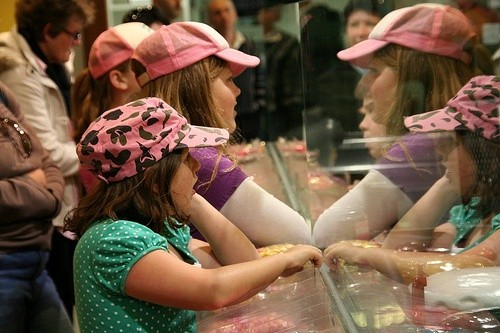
[0, 117, 32, 158]
[61, 26, 81, 41]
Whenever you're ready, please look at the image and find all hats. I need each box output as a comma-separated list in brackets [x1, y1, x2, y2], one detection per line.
[338, 4, 478, 70]
[131, 21, 259, 87]
[404, 74, 500, 143]
[87, 22, 156, 80]
[76, 97, 229, 193]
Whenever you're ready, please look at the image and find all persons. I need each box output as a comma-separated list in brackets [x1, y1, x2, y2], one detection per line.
[68, 0, 268, 150]
[249, 0, 499, 164]
[0, 0, 88, 327]
[129, 20, 311, 246]
[0, 94, 75, 332]
[248, 0, 500, 293]
[60, 96, 324, 333]
